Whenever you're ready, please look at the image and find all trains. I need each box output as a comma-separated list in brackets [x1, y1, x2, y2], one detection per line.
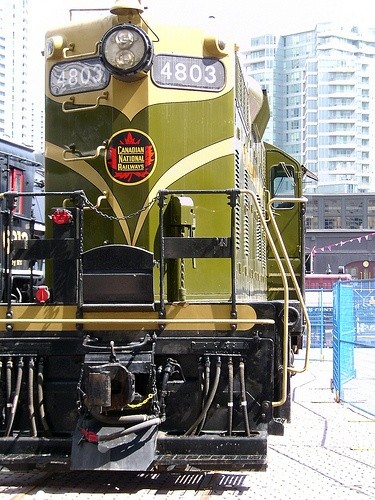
[2, 0, 321, 476]
